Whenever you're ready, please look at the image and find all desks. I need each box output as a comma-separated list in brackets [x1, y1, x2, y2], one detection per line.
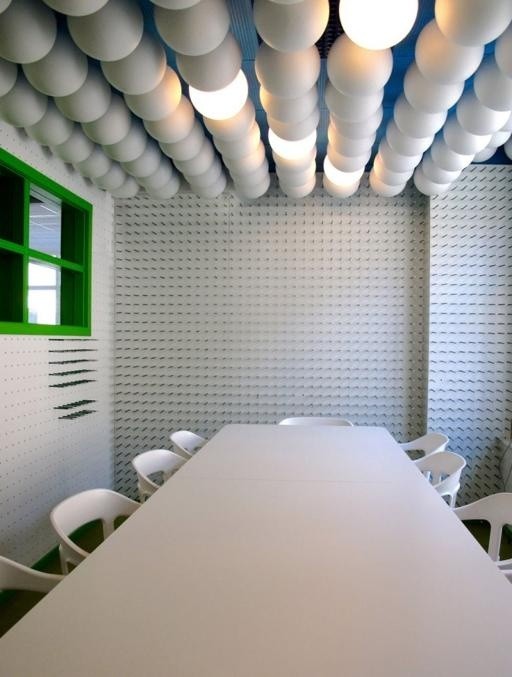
[0, 425, 512, 677]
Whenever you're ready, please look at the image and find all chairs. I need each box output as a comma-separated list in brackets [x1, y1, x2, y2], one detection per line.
[170, 430, 207, 461]
[280, 416, 352, 427]
[454, 492, 512, 581]
[398, 432, 450, 461]
[132, 450, 186, 505]
[0, 554, 68, 636]
[51, 487, 143, 576]
[417, 450, 467, 506]
